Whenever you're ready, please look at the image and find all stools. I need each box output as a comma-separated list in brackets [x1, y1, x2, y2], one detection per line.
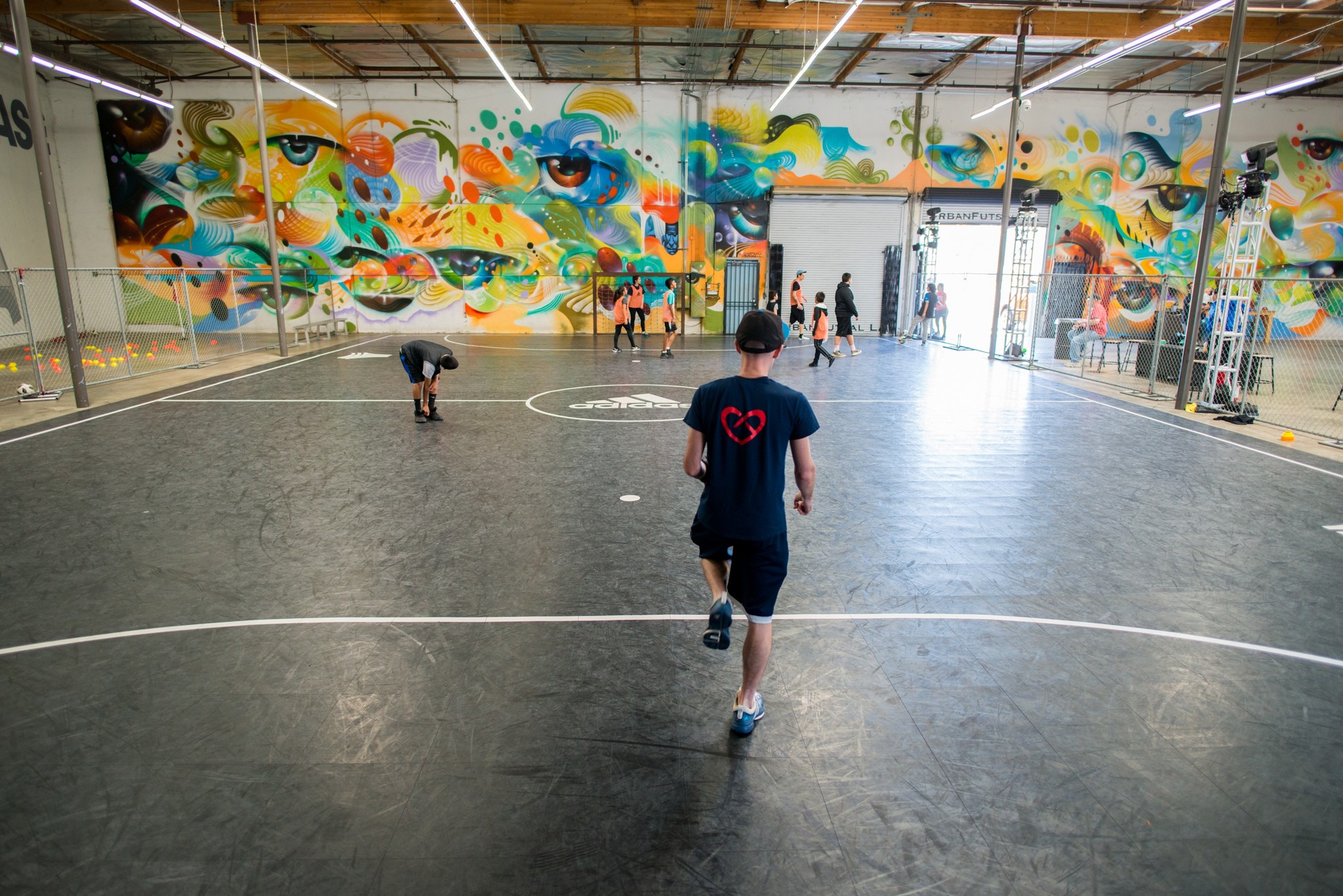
[1097, 338, 1123, 374]
[1121, 339, 1148, 372]
[1081, 337, 1105, 368]
[1241, 353, 1274, 393]
[1175, 360, 1208, 401]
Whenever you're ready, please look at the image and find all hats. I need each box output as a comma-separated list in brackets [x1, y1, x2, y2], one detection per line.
[797, 270, 806, 276]
[736, 310, 790, 353]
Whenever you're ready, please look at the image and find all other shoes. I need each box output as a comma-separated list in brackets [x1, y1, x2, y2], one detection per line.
[942, 334, 946, 339]
[783, 345, 787, 348]
[933, 332, 940, 336]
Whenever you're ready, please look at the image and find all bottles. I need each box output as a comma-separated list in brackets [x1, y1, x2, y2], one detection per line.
[1203, 340, 1208, 353]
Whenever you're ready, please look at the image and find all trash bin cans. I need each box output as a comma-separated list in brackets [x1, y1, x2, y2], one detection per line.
[1053, 317, 1085, 359]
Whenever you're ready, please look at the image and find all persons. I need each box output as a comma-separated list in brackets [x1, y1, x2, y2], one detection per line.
[832, 272, 862, 357]
[683, 309, 821, 734]
[809, 292, 835, 367]
[612, 286, 640, 351]
[1181, 282, 1214, 332]
[1203, 288, 1245, 366]
[660, 278, 678, 358]
[787, 270, 810, 340]
[399, 340, 459, 422]
[902, 283, 943, 345]
[627, 276, 651, 337]
[934, 283, 948, 339]
[1064, 293, 1107, 368]
[766, 291, 788, 349]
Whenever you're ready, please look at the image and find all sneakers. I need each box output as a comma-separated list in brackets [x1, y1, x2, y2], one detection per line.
[413, 409, 427, 422]
[630, 345, 640, 351]
[852, 349, 862, 356]
[809, 363, 818, 366]
[667, 350, 674, 358]
[641, 332, 650, 337]
[632, 332, 634, 337]
[829, 357, 835, 367]
[832, 351, 846, 357]
[1064, 361, 1081, 368]
[702, 590, 732, 650]
[731, 687, 764, 733]
[613, 346, 621, 352]
[424, 407, 443, 420]
[922, 341, 925, 345]
[660, 351, 671, 358]
[798, 336, 810, 340]
[902, 336, 912, 340]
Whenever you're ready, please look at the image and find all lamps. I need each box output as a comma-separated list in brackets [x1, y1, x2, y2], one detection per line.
[971, 0, 1343, 120]
[1238, 141, 1278, 181]
[1019, 187, 1041, 211]
[451, 0, 533, 111]
[913, 207, 942, 252]
[769, 0, 863, 111]
[0, 0, 338, 109]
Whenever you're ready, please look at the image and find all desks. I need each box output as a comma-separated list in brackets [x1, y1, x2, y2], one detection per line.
[1249, 310, 1276, 345]
[1135, 342, 1216, 391]
